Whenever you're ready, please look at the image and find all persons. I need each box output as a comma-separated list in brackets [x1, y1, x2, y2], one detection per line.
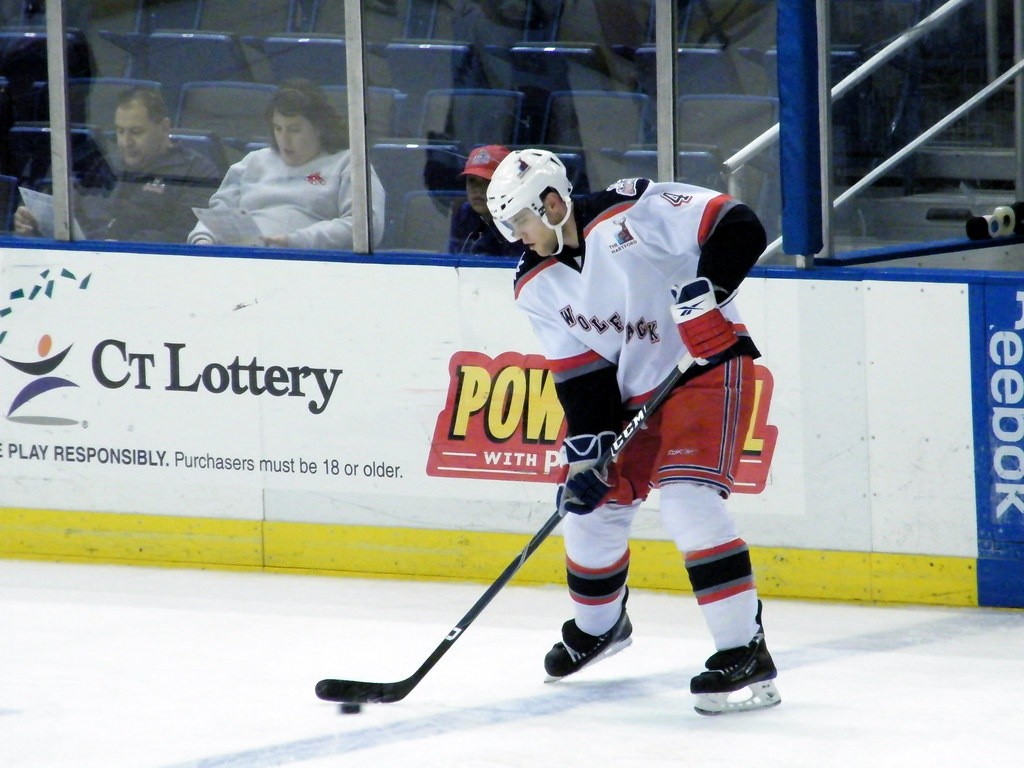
[186, 78, 384, 251]
[486, 149, 777, 693]
[14, 87, 220, 243]
[448, 145, 526, 256]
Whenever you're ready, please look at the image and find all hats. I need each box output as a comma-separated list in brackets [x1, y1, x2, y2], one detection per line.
[460, 145, 512, 180]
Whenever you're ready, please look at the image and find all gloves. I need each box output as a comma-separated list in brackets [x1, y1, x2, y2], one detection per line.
[669, 277, 740, 366]
[556, 430, 617, 518]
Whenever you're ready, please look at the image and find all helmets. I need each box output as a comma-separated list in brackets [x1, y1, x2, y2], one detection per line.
[486, 148, 573, 243]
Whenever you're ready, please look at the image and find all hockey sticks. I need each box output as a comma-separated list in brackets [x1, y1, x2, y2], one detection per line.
[313, 314, 734, 706]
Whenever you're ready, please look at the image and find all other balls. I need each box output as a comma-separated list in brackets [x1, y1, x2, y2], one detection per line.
[339, 703, 361, 714]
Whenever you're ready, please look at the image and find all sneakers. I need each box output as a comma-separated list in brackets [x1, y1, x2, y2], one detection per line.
[542, 584, 634, 683]
[688, 599, 781, 715]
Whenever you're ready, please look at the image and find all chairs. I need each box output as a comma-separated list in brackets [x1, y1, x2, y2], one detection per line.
[0, 0, 1007, 267]
